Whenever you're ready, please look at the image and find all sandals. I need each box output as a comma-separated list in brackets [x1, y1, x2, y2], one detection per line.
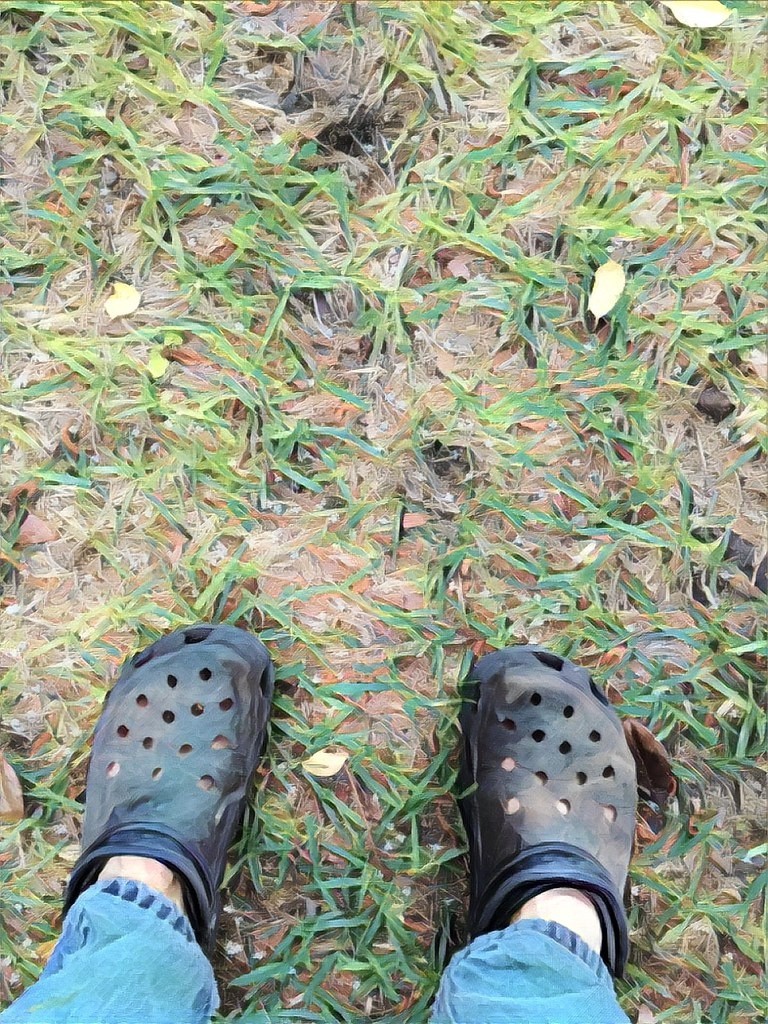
[61, 624, 275, 956]
[456, 646, 639, 978]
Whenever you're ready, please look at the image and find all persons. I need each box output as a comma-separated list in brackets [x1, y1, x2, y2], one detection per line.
[0, 623, 639, 1024]
